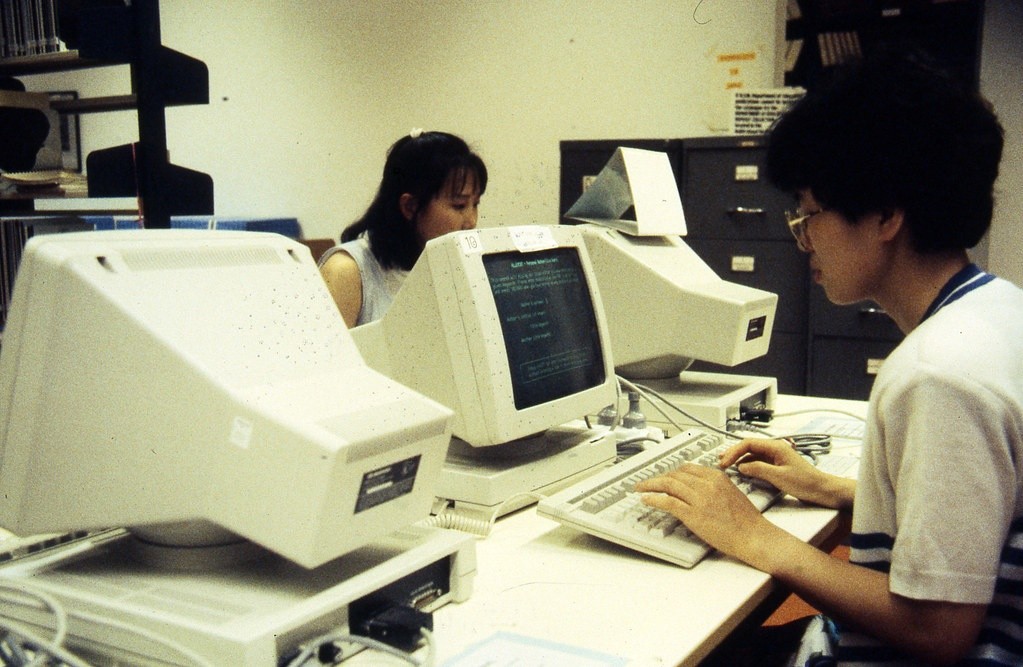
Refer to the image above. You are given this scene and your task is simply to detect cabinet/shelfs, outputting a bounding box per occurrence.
[556,137,908,404]
[0,1,217,233]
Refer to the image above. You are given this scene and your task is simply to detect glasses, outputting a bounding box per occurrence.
[785,206,829,252]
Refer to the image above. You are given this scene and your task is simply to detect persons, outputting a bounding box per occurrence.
[632,45,1022,666]
[315,128,489,331]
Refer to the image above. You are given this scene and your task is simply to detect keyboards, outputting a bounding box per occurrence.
[535,428,819,567]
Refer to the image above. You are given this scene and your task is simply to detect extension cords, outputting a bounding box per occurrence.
[562,420,663,453]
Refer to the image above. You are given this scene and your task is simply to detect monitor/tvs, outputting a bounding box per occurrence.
[3,228,451,562]
[347,225,618,443]
[569,223,777,380]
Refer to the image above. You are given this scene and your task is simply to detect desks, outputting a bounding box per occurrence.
[322,390,870,667]
